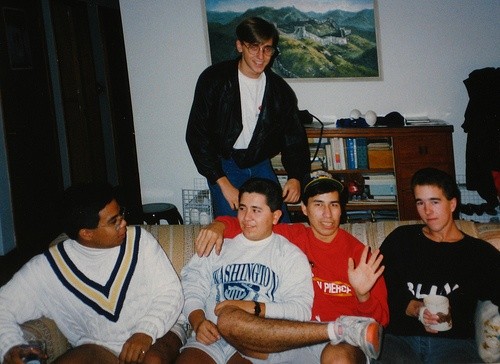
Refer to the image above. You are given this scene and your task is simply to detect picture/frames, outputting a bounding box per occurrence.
[201,0,382,81]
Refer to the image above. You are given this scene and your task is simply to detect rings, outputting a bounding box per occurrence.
[142,351,145,353]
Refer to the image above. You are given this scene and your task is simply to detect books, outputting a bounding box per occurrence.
[270,137,369,171]
[406,116,429,123]
[362,172,397,200]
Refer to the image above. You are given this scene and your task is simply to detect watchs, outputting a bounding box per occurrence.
[253,301,261,316]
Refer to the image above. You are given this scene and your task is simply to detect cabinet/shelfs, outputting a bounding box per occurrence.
[270,119,458,224]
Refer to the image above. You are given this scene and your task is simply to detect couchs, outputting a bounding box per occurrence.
[21,220,500,363]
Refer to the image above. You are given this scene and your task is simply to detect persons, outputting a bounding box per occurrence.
[0,180,184,364]
[186,17,311,224]
[371,166,500,364]
[195,176,391,364]
[180,178,314,364]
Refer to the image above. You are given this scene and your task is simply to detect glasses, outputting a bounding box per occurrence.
[243,42,275,57]
[86,209,127,232]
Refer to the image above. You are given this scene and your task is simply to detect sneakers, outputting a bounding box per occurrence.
[330,315,383,360]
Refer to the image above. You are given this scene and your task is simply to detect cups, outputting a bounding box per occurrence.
[424,295,452,331]
[19,340,47,364]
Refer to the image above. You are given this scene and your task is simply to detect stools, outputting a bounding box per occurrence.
[133,203,184,225]
